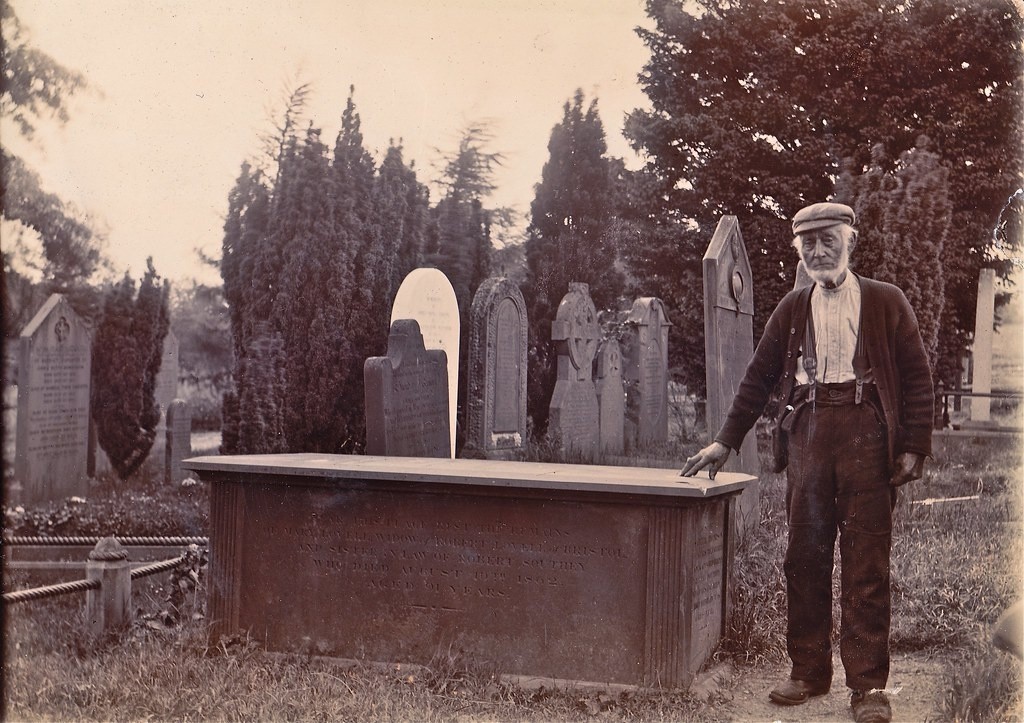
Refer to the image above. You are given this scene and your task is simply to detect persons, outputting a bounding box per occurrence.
[679,201,936,723]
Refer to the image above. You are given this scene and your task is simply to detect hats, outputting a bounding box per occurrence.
[792,202,855,236]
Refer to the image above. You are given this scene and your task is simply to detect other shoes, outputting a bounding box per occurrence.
[847,689,892,723]
[769,678,829,705]
